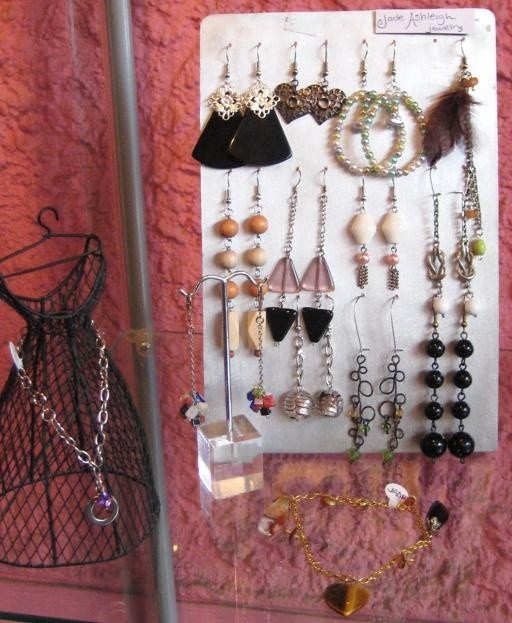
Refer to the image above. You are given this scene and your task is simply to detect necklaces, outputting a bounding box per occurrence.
[9,318,119,525]
[290,490,441,617]
